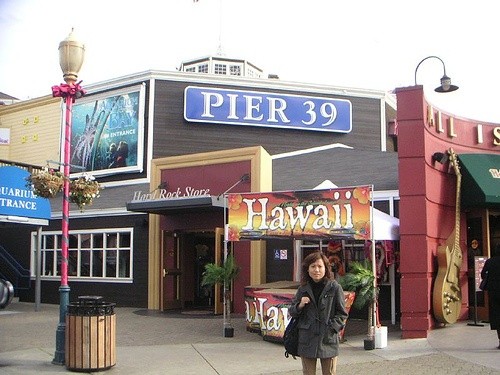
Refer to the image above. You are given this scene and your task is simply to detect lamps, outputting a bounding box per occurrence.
[414,55,459,93]
[432,151,449,164]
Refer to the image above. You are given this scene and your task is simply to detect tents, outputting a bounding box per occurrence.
[312,179,400,325]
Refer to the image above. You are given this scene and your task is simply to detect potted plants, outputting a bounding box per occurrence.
[199,253,242,338]
[338,257,380,350]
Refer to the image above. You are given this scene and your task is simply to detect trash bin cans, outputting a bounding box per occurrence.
[65,296,116,373]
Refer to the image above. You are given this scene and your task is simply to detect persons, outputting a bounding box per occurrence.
[288,250,348,375]
[468,239,484,306]
[479,242,500,350]
[107,141,128,168]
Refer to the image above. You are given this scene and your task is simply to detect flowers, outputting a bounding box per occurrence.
[25,168,101,210]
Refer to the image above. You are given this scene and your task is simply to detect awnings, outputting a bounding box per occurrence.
[456,154,500,203]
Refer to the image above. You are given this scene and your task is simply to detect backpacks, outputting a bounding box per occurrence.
[283,288,307,357]
